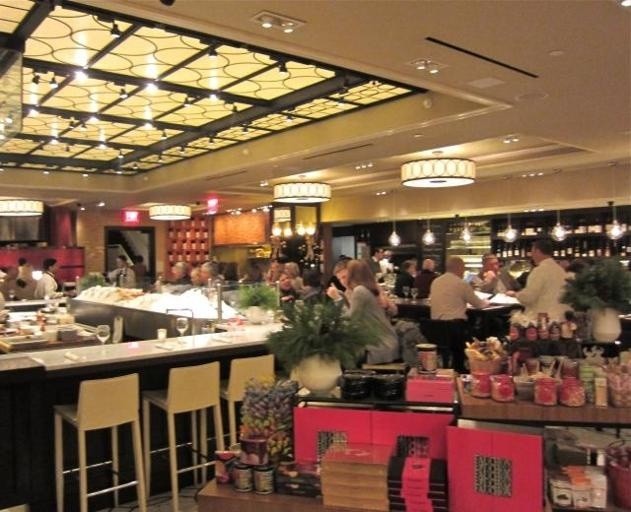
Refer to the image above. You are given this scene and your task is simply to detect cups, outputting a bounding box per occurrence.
[157,328,167,344]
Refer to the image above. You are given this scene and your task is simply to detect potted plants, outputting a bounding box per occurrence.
[229,282,279,324]
[266,287,393,395]
[558,256,631,345]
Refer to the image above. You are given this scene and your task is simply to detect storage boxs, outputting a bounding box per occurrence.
[405,375,457,404]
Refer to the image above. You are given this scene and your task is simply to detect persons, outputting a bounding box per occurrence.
[427,257,492,375]
[104,255,147,283]
[329,258,399,323]
[503,239,569,325]
[326,258,401,375]
[469,252,536,293]
[163,260,239,289]
[0,256,75,309]
[237,258,322,305]
[558,258,588,275]
[378,257,441,299]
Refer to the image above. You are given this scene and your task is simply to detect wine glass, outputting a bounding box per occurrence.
[175,318,189,343]
[227,315,241,337]
[410,288,419,304]
[95,324,111,354]
[261,309,287,332]
[402,286,410,301]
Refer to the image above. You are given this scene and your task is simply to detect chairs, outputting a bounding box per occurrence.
[201,352,280,487]
[51,371,142,512]
[140,360,224,510]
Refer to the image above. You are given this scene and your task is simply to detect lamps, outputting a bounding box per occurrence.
[400,157,477,189]
[460,218,474,244]
[605,162,626,241]
[405,57,450,75]
[273,182,332,206]
[421,192,436,247]
[502,176,518,243]
[0,199,46,217]
[388,188,403,247]
[149,205,192,222]
[550,166,568,242]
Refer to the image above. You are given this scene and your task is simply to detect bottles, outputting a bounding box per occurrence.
[495,219,631,235]
[471,355,610,408]
[448,222,491,232]
[494,239,631,258]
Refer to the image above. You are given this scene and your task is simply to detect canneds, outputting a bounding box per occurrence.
[233,462,253,493]
[253,464,276,495]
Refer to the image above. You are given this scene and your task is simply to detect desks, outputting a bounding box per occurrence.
[457,376,631,429]
[1,297,69,313]
[196,476,383,511]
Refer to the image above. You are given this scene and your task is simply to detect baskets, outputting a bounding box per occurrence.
[468,352,507,375]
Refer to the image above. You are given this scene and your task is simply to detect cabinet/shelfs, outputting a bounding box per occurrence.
[492,205,630,268]
